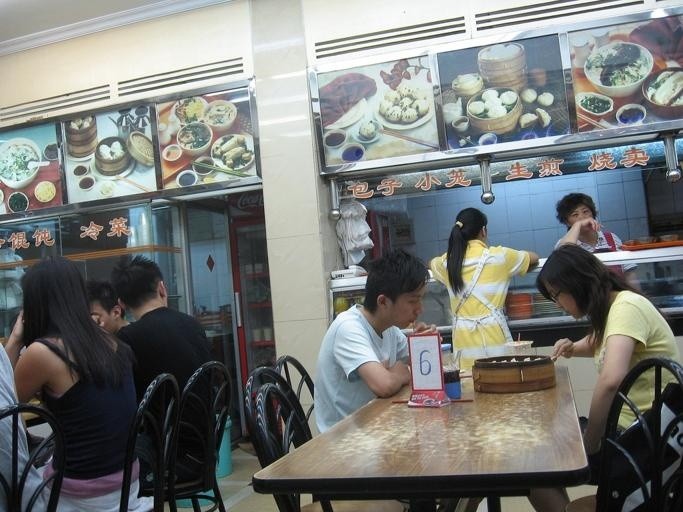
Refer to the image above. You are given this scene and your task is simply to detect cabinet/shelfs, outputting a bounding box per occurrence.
[338,243,681,349]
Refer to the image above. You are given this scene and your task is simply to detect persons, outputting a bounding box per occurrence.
[527,243,682,512]
[114,254,209,484]
[83,280,131,336]
[553,192,642,342]
[427,207,539,372]
[4,259,142,511]
[0,342,43,512]
[312,248,443,433]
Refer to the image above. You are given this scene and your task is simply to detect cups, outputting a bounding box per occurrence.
[573,36,588,64]
[503,340,532,356]
[591,27,609,48]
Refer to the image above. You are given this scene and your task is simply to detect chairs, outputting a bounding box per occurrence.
[569,353,682,512]
[267,349,322,443]
[252,377,406,512]
[106,370,181,512]
[240,354,333,512]
[128,348,234,512]
[0,399,65,511]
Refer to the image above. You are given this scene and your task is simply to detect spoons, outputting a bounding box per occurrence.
[24,159,51,170]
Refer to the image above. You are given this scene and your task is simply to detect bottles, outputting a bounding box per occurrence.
[168,115,179,136]
[158,122,171,147]
[438,344,461,398]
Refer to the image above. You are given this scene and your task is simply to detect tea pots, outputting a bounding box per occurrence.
[107,107,133,141]
[442,96,461,126]
[128,106,151,140]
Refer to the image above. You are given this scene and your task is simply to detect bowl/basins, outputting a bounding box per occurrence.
[613,103,645,124]
[621,232,678,244]
[201,101,236,129]
[452,114,469,131]
[0,137,41,189]
[176,122,212,153]
[477,131,496,146]
[582,41,653,96]
[521,133,535,140]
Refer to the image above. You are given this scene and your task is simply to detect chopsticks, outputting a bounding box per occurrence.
[114,173,152,193]
[381,130,440,150]
[188,160,250,177]
[458,135,474,146]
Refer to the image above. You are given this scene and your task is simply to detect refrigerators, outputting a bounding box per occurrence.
[226,191,392,439]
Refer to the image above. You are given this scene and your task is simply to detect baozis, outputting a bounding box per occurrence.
[451,43,555,128]
[70,115,93,130]
[98,141,125,161]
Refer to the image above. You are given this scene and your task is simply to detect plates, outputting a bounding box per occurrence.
[91,155,136,181]
[70,163,89,177]
[192,156,214,175]
[374,101,433,131]
[351,119,381,144]
[33,180,56,203]
[175,169,197,187]
[169,95,207,123]
[6,191,30,214]
[65,152,95,162]
[209,133,253,171]
[322,97,367,130]
[161,144,181,161]
[76,176,96,191]
[42,142,59,162]
[336,142,366,162]
[323,127,348,149]
[575,91,613,116]
